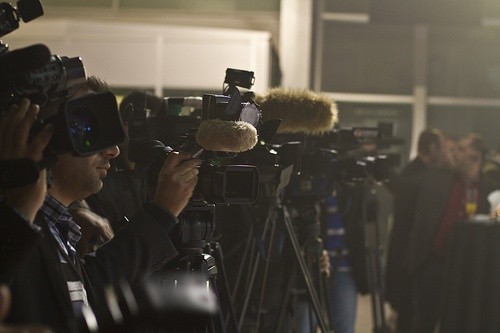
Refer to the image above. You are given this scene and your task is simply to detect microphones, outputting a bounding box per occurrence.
[254,87,339,133]
[187,118,259,154]
[1,43,51,85]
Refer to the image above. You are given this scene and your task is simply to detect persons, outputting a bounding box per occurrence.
[0,74,500,333]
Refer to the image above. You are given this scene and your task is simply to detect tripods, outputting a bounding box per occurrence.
[178,177,390,333]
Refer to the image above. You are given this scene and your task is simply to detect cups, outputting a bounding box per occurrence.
[465,189,477,217]
